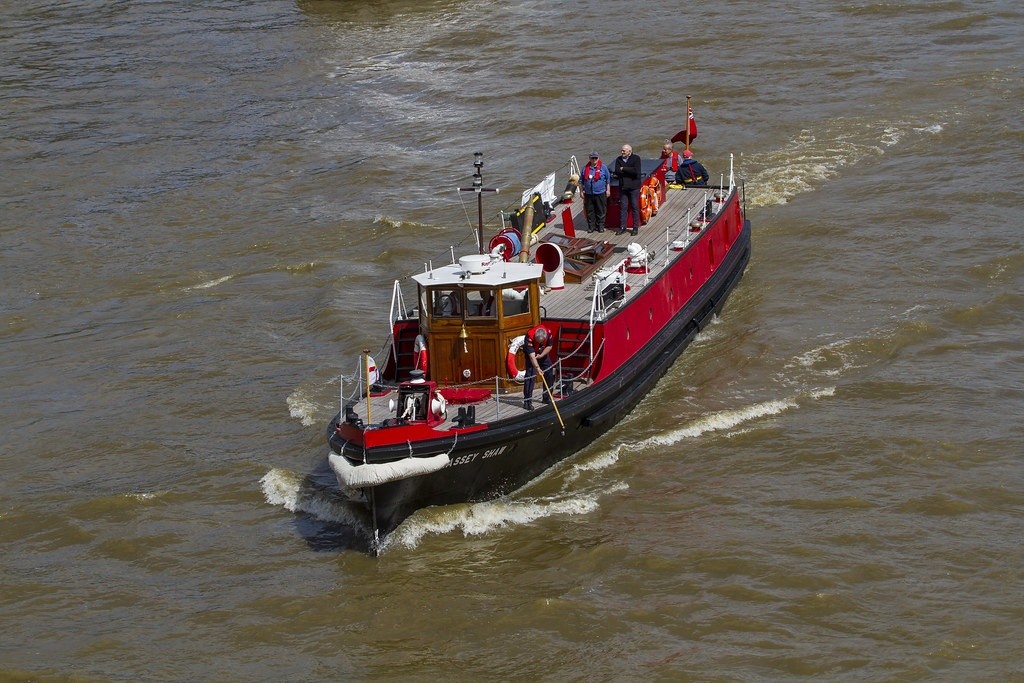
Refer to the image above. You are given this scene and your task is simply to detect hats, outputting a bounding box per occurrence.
[589,151,599,157]
[683,150,693,158]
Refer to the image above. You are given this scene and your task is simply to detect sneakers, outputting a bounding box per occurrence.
[542,398,552,404]
[524,403,534,410]
[595,225,604,233]
[588,226,596,233]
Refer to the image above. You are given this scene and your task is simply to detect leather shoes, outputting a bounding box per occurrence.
[631,228,638,236]
[615,226,626,235]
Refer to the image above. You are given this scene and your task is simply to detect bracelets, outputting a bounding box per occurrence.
[535,365,539,369]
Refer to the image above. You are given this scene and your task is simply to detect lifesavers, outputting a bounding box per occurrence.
[413,334,428,379]
[506,335,527,381]
[637,176,663,225]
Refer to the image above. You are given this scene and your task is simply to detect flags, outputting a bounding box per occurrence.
[672,107,697,145]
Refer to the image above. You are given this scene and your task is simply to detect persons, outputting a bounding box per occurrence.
[476,291,493,316]
[444,291,475,315]
[615,145,642,235]
[580,152,610,231]
[676,152,708,185]
[523,325,553,411]
[660,142,681,177]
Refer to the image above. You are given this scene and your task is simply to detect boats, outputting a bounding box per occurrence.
[326,93,752,556]
